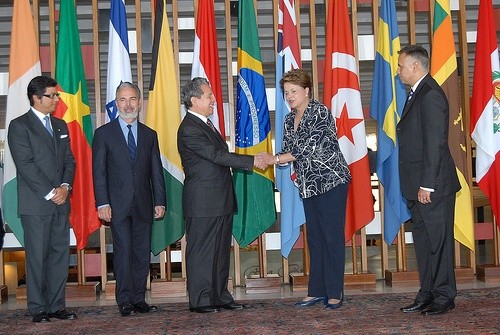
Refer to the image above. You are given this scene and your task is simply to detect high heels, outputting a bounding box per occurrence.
[324,297,343,310]
[294,296,327,308]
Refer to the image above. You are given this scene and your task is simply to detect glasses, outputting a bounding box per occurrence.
[42,92,59,99]
[116,97,138,103]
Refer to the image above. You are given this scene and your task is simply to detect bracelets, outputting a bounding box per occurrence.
[277,154,280,165]
[63,184,72,190]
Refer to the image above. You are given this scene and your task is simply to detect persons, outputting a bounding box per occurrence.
[176,77,269,313]
[256,67,353,310]
[8,76,78,322]
[90,80,168,319]
[396,46,462,316]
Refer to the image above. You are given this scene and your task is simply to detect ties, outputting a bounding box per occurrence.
[127,125,136,166]
[44,116,53,138]
[207,119,218,136]
[405,89,414,107]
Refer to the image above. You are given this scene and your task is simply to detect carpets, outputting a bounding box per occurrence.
[0,285,500,335]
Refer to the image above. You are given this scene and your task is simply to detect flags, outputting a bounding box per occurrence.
[372,0,411,245]
[0,0,78,248]
[104,0,133,125]
[143,0,187,256]
[324,0,374,243]
[470,0,500,229]
[191,0,226,140]
[52,0,102,250]
[429,0,475,253]
[271,0,306,260]
[233,0,278,249]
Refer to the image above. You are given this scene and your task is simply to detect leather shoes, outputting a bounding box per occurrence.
[32,312,50,323]
[48,309,76,320]
[400,299,429,313]
[421,302,456,316]
[213,301,246,311]
[189,305,223,313]
[135,301,157,314]
[120,305,138,317]
